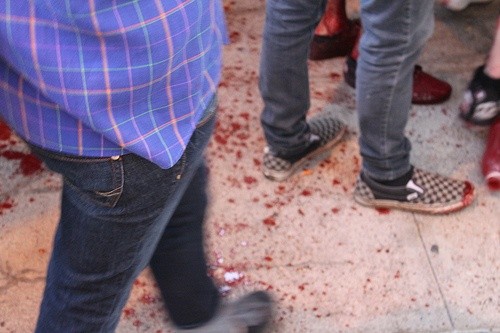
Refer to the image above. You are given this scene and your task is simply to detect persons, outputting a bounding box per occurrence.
[0,0,275,333]
[259,0,500,213]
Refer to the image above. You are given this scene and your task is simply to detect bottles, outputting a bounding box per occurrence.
[481,117,500,191]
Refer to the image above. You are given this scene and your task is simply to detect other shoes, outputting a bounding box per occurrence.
[457,64,500,126]
[170,289,274,333]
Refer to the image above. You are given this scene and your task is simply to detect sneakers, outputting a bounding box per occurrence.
[261,116,346,183]
[342,54,452,105]
[308,17,361,61]
[353,165,476,215]
